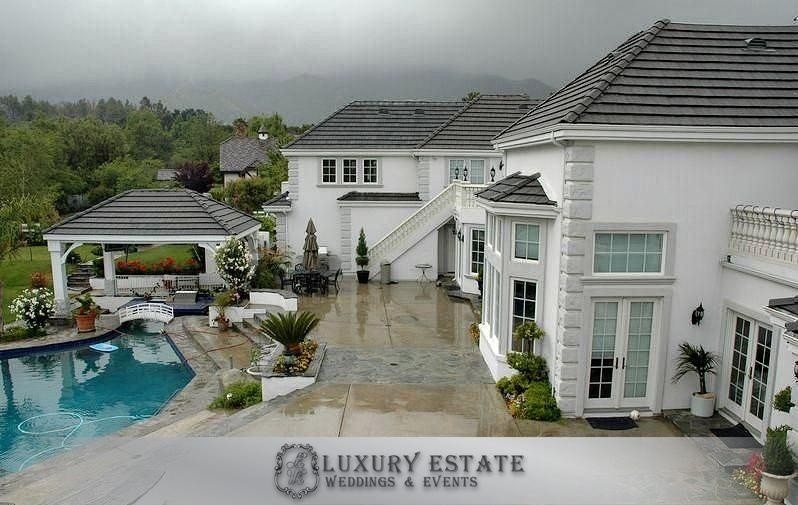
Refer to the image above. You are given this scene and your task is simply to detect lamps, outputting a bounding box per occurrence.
[691,302,704,326]
[793,360,798,384]
[463,166,468,180]
[454,166,459,180]
[490,165,495,182]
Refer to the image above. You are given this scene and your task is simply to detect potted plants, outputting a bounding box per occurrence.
[257,310,320,356]
[355,225,369,284]
[71,293,101,333]
[669,341,721,418]
[209,289,232,332]
[754,425,797,505]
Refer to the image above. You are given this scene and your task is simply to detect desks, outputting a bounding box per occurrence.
[415,265,432,285]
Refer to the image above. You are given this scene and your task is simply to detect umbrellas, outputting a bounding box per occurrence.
[303,218,319,270]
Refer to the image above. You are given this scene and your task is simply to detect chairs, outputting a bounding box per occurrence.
[291,262,341,296]
